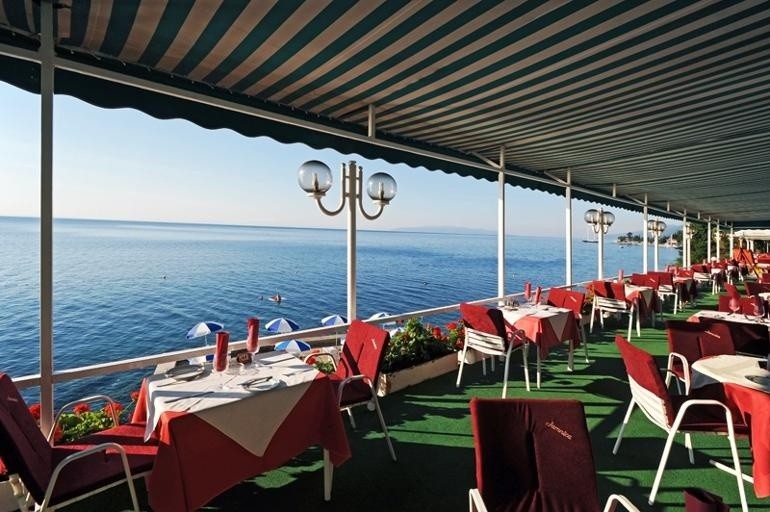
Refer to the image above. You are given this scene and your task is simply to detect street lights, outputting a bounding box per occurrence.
[686,221,698,268]
[583,207,617,280]
[647,218,667,275]
[297,158,399,326]
[712,229,726,263]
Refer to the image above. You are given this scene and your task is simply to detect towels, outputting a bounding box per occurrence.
[216,316,260,371]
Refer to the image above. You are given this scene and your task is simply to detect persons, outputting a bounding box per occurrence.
[275,293,281,302]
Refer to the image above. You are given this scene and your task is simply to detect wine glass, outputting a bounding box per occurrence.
[212,352,229,380]
[525,294,532,307]
[753,304,766,324]
[245,335,260,369]
[729,299,741,319]
[674,271,680,278]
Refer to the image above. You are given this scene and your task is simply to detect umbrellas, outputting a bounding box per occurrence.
[274,339,311,352]
[369,311,396,330]
[265,317,299,333]
[321,314,348,346]
[189,354,214,365]
[186,320,224,346]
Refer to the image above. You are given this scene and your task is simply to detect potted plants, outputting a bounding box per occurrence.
[373,313,461,398]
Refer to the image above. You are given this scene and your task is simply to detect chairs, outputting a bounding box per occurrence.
[304,317,400,464]
[0,371,159,512]
[455,247,769,512]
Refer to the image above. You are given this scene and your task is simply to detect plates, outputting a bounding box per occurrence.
[743,373,770,390]
[242,375,280,392]
[164,364,205,382]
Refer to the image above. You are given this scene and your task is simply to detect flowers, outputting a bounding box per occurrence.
[432,316,470,351]
[28,388,142,445]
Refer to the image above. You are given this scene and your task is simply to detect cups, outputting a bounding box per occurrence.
[534,298,541,308]
